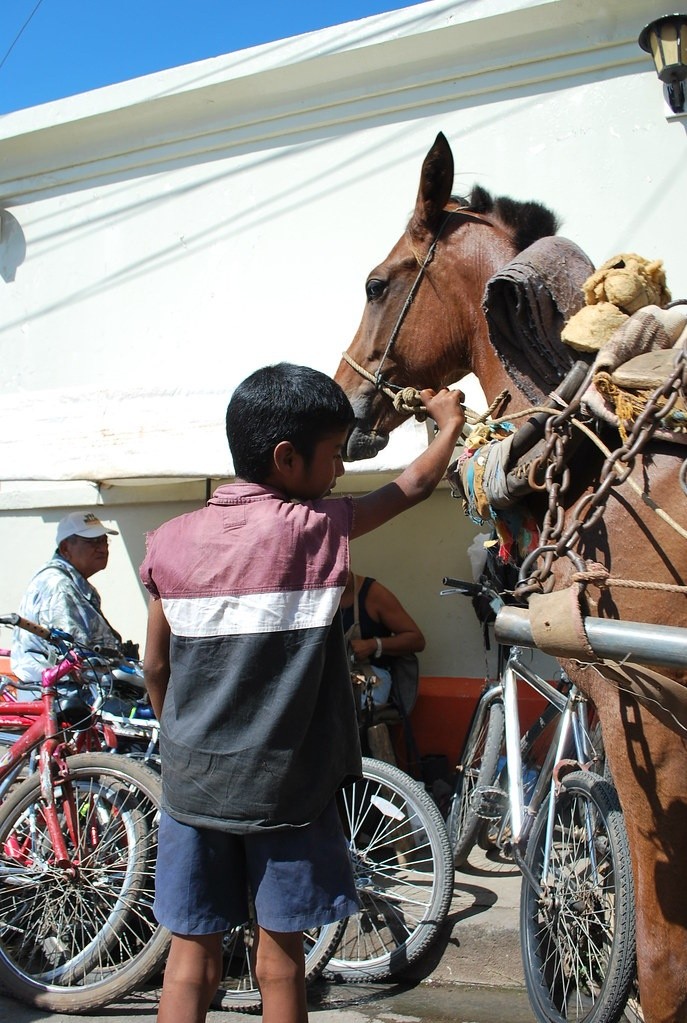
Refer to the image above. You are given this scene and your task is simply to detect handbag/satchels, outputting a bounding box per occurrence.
[341,622,381,685]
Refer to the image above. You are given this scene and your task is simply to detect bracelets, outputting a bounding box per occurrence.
[373,637,382,658]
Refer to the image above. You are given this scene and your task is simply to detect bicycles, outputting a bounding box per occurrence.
[431,543,648,1023]
[0,613,452,1015]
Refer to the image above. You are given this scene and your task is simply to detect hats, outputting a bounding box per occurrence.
[57,512,119,547]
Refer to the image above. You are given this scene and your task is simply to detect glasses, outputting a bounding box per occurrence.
[71,536,109,545]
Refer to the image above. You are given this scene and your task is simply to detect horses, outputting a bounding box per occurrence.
[330,131,687,1023]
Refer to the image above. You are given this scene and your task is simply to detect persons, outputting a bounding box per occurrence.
[140,363,465,1023]
[338,567,426,866]
[10,511,161,829]
